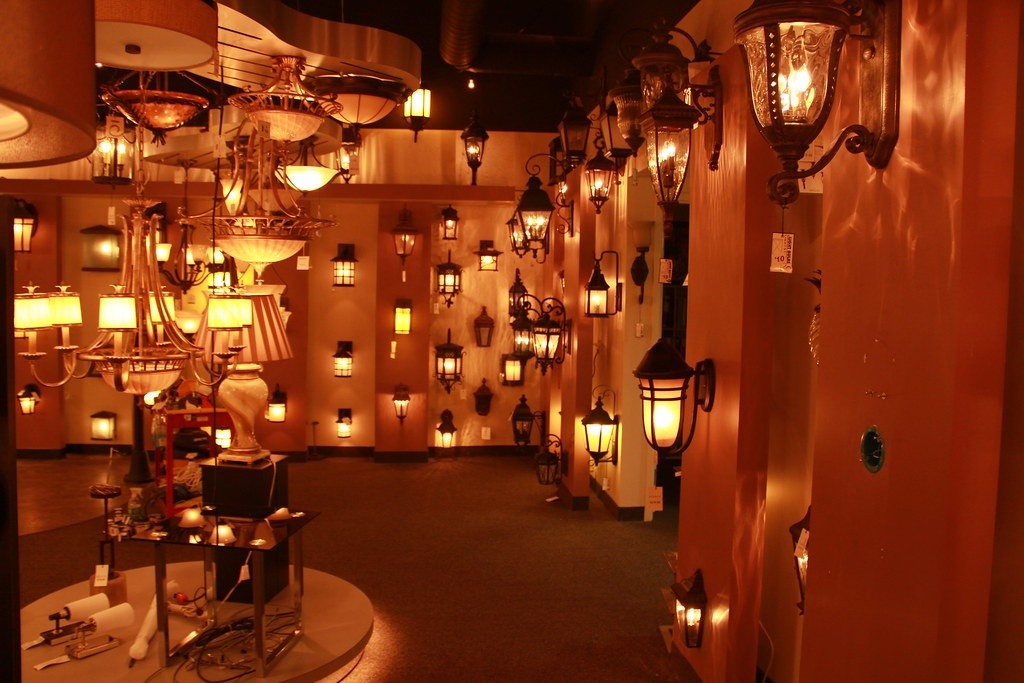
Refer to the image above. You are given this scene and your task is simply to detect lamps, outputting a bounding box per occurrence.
[0,0,899,683]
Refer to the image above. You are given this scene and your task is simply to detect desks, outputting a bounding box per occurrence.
[100,492,319,679]
[20,562,375,683]
